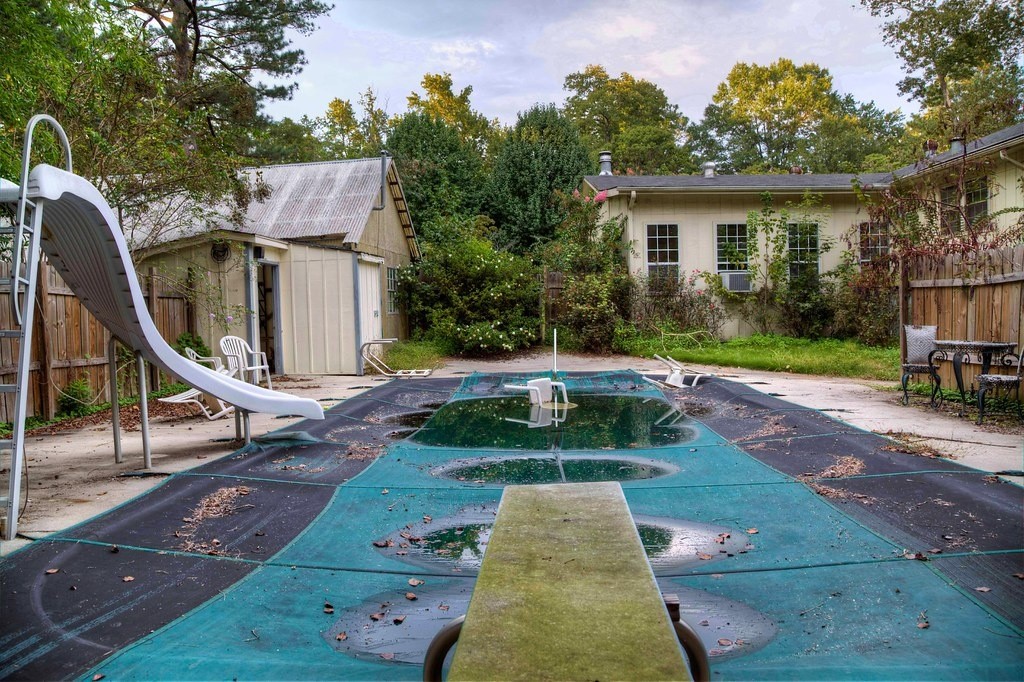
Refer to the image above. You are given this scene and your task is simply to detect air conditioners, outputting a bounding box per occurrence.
[718,272,752,293]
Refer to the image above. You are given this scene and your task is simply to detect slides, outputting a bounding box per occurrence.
[0,164,326,421]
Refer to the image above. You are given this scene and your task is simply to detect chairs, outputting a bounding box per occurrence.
[505,405,568,428]
[642,353,712,388]
[185,346,224,402]
[900,324,941,407]
[503,376,578,410]
[220,336,273,390]
[642,399,684,426]
[973,344,1024,426]
[158,364,239,421]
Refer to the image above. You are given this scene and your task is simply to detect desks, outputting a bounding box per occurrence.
[926,339,1018,416]
